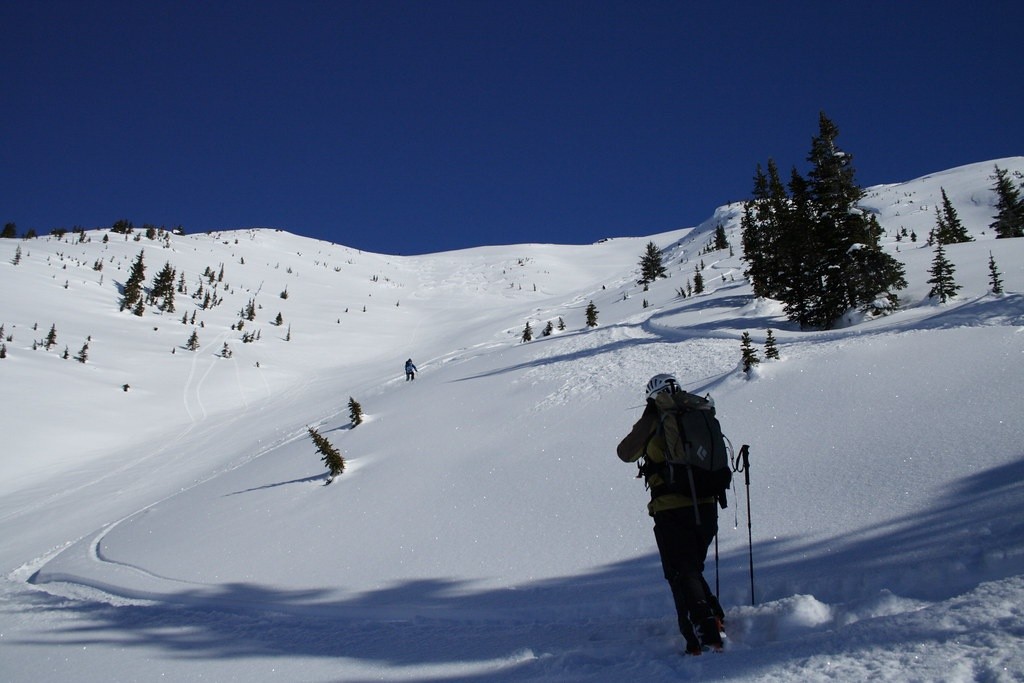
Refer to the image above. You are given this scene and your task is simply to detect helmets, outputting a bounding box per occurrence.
[645,373,681,403]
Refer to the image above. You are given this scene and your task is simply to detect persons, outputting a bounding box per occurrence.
[617,373,732,658]
[405,359,417,381]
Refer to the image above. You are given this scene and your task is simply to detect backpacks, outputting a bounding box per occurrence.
[645,391,733,509]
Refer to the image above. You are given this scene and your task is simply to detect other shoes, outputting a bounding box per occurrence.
[715,615,726,638]
[682,642,724,657]
